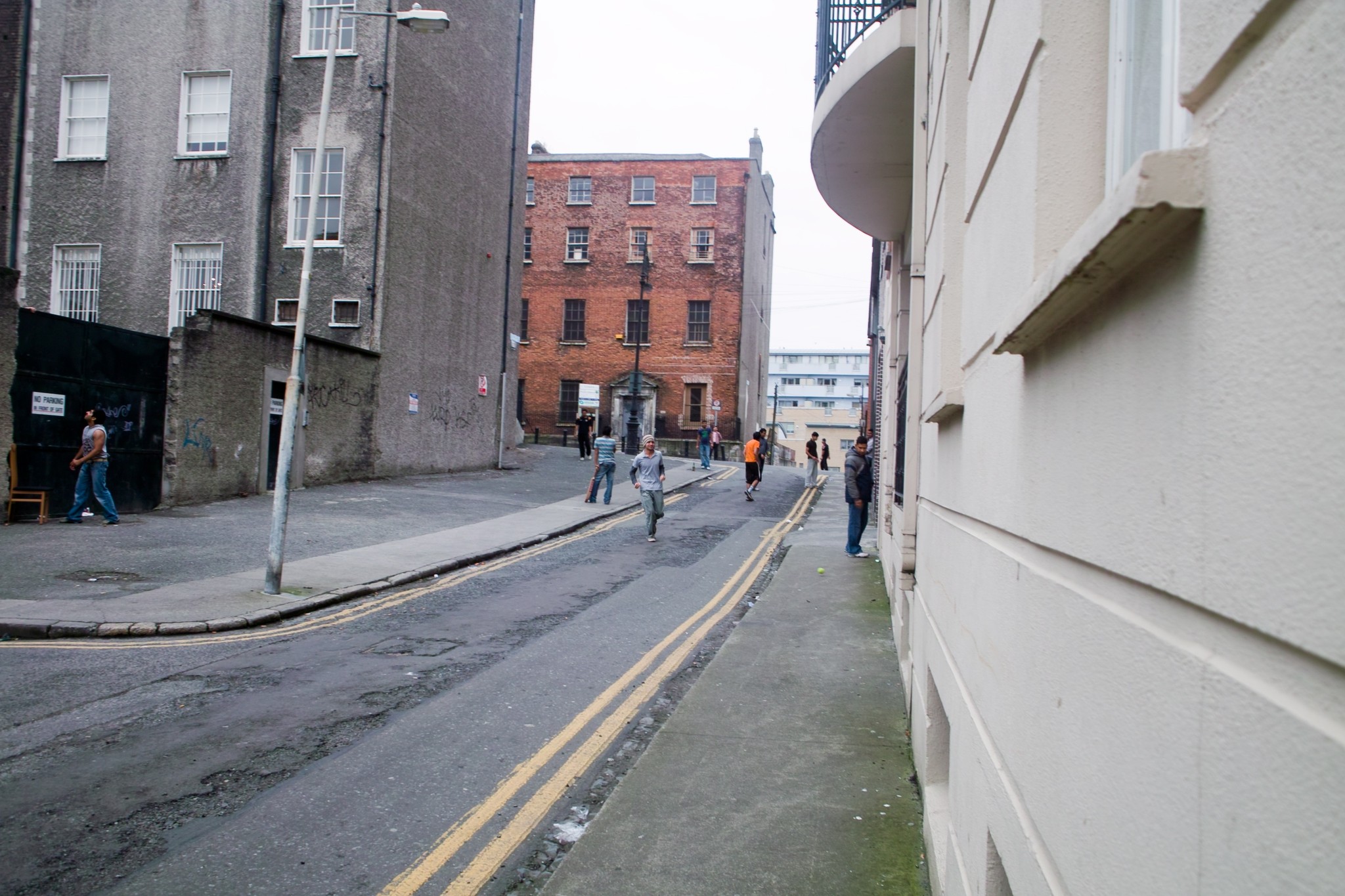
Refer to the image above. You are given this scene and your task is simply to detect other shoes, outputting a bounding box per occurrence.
[707,466,710,470]
[101,519,120,525]
[648,537,656,542]
[588,455,592,460]
[581,457,584,460]
[804,485,813,488]
[60,518,83,524]
[754,488,760,491]
[744,491,754,501]
[812,485,819,488]
[701,466,705,469]
[844,548,870,557]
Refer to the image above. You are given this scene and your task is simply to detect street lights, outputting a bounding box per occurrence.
[263,5,456,596]
[624,232,655,456]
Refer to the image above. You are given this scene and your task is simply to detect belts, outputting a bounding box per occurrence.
[88,458,108,463]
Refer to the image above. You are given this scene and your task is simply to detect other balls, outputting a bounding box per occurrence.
[818,568,825,574]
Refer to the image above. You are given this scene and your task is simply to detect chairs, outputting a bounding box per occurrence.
[3,443,52,526]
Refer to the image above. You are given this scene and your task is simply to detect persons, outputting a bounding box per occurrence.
[59,408,121,525]
[574,409,593,460]
[806,432,819,488]
[820,439,831,471]
[845,435,873,557]
[865,429,874,469]
[750,428,770,491]
[743,431,761,502]
[696,420,713,470]
[584,426,617,505]
[710,426,723,461]
[630,434,666,542]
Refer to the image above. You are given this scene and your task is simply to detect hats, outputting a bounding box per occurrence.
[643,434,656,448]
